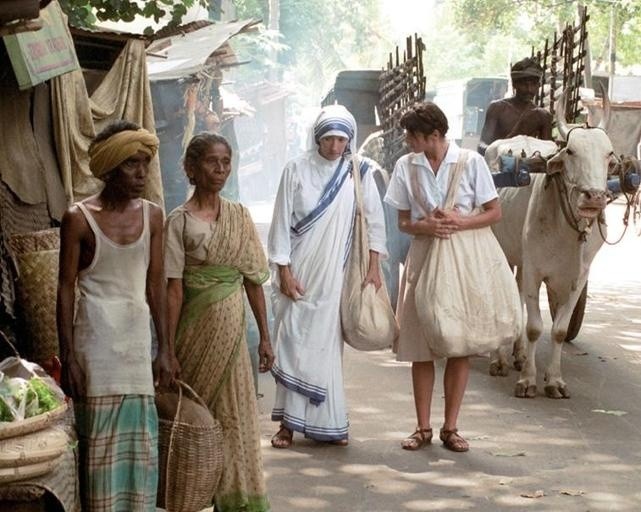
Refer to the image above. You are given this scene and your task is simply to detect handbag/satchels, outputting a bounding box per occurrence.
[415,203,525,358]
[334,214,403,356]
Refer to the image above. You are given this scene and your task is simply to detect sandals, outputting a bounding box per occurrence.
[439,427,470,452]
[401,426,433,450]
[271,424,293,448]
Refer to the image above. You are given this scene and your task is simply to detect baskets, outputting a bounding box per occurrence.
[152,377,224,512]
[8,228,75,360]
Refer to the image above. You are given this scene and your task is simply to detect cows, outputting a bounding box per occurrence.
[476,80,613,399]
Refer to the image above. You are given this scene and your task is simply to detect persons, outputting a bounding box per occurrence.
[55,119,176,512]
[476,55,554,159]
[161,132,277,512]
[381,100,504,453]
[266,104,391,448]
